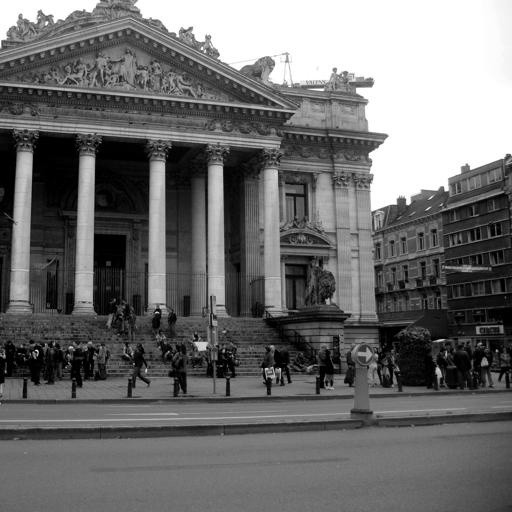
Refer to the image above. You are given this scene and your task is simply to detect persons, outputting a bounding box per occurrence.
[260,346,314,385]
[342,70,353,92]
[318,346,356,389]
[105,299,178,339]
[304,257,318,305]
[123,343,152,387]
[366,347,402,392]
[0,339,110,387]
[329,67,340,92]
[15,8,223,99]
[424,341,511,390]
[157,330,238,395]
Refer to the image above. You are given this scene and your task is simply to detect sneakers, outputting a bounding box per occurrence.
[325,385,335,390]
